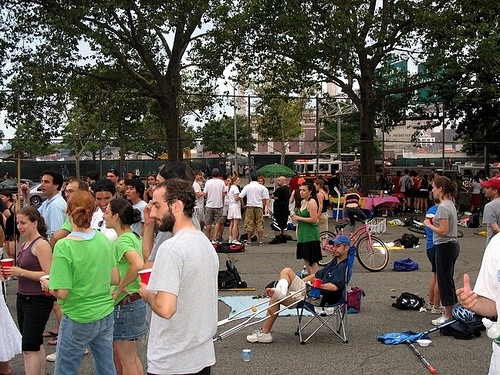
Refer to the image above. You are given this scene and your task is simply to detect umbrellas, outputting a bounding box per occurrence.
[257,163,296,192]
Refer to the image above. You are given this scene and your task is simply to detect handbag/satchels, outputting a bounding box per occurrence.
[218,259,247,289]
[392,291,425,310]
[346,286,366,314]
[213,243,246,252]
[439,317,481,339]
[397,233,419,248]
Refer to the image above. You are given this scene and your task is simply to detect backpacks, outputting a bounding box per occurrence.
[393,258,418,271]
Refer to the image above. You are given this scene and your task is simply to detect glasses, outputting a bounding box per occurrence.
[96,219,103,231]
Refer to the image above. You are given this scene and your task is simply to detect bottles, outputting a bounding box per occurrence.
[391,289,396,298]
[481,317,500,347]
[300,267,308,278]
[289,207,299,226]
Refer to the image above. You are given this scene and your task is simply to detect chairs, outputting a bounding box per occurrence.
[295,246,358,344]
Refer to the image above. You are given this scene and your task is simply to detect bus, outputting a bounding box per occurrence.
[291,159,343,181]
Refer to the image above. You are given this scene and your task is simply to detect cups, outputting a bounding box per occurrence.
[426,214,435,225]
[243,349,251,361]
[313,279,323,288]
[41,274,53,296]
[0,258,14,277]
[138,269,152,285]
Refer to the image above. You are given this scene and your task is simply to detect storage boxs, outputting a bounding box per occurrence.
[333,208,347,218]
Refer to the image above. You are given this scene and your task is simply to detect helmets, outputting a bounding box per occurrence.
[452,304,476,320]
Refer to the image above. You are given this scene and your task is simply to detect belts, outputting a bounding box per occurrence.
[117,293,143,305]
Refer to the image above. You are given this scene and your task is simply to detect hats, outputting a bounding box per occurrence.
[480,177,500,190]
[329,235,350,245]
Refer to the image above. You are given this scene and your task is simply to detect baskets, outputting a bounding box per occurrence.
[367,217,386,235]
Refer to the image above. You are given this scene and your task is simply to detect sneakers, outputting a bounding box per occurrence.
[246,329,273,342]
[211,231,288,246]
[426,302,435,309]
[431,315,450,326]
[431,304,446,314]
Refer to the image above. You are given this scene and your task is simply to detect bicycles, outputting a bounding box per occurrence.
[317,212,389,272]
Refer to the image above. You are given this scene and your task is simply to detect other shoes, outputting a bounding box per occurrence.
[399,208,423,213]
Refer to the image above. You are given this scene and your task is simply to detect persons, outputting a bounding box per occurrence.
[0,158,500,375]
[246,234,351,344]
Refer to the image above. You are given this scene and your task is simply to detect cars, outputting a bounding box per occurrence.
[0,178,34,193]
[29,182,48,206]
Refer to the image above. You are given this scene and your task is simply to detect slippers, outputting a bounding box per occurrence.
[43,329,89,361]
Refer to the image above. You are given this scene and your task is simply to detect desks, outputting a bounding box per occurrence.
[330,196,399,220]
